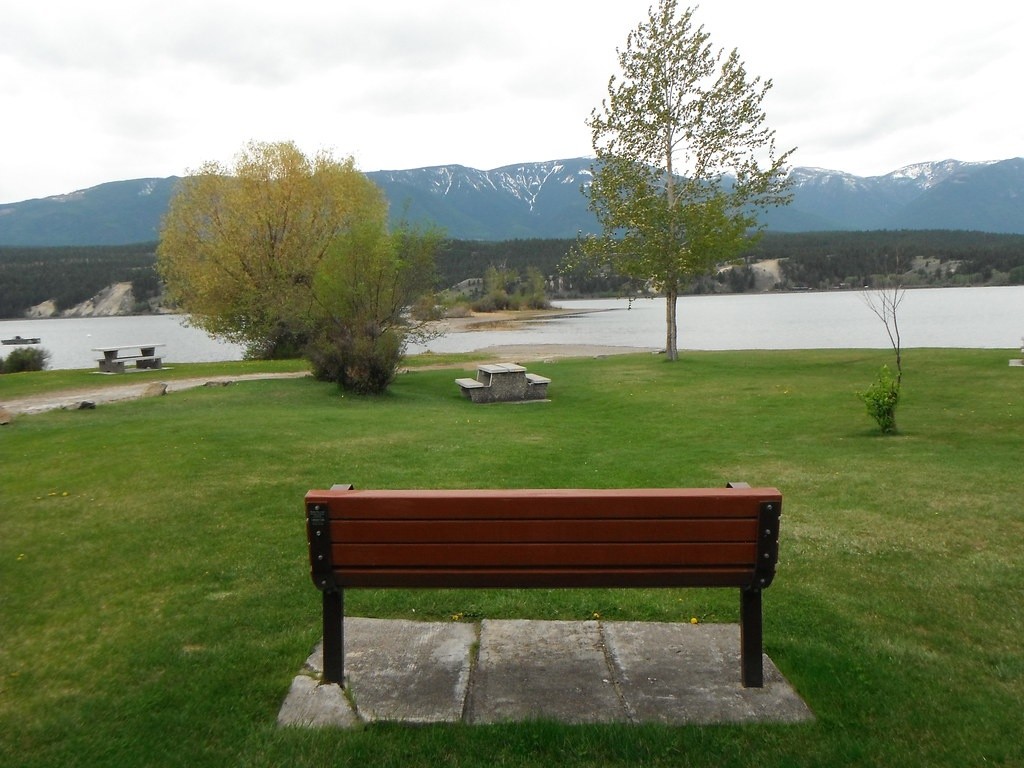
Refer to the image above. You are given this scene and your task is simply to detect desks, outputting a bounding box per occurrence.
[91,344,167,373]
[476,363,527,403]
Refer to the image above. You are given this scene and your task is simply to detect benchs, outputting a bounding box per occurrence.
[96,356,167,373]
[455,378,485,403]
[305,481,783,690]
[525,374,552,401]
